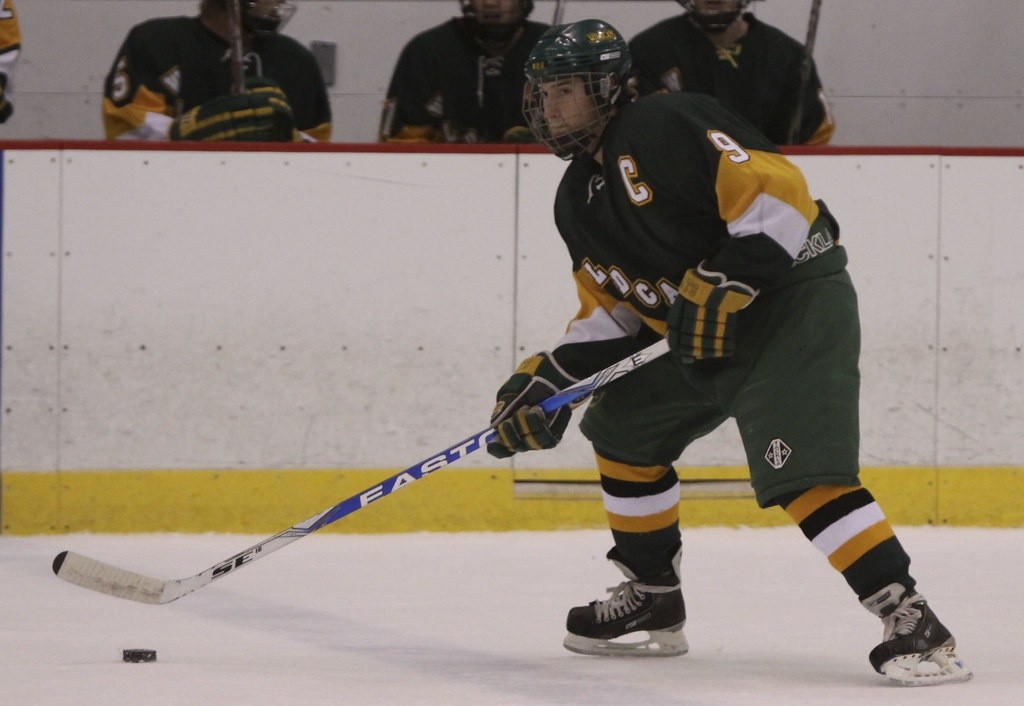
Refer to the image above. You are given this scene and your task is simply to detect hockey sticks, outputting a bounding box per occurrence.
[787,0,823,146]
[226,0,246,96]
[49,309,759,607]
[551,0,565,28]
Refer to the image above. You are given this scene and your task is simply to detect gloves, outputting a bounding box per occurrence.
[665,271,754,373]
[167,82,295,140]
[485,350,581,462]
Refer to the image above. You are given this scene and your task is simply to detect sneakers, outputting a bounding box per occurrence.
[856,579,972,683]
[563,548,689,656]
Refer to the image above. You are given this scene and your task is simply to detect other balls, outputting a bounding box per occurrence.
[121,648,157,664]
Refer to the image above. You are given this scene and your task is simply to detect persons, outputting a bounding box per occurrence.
[627,0,836,145]
[376,0,553,143]
[485,17,965,684]
[102,0,333,142]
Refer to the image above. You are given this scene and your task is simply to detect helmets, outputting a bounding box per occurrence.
[676,0,751,33]
[522,20,635,160]
[239,0,297,36]
[460,0,534,39]
